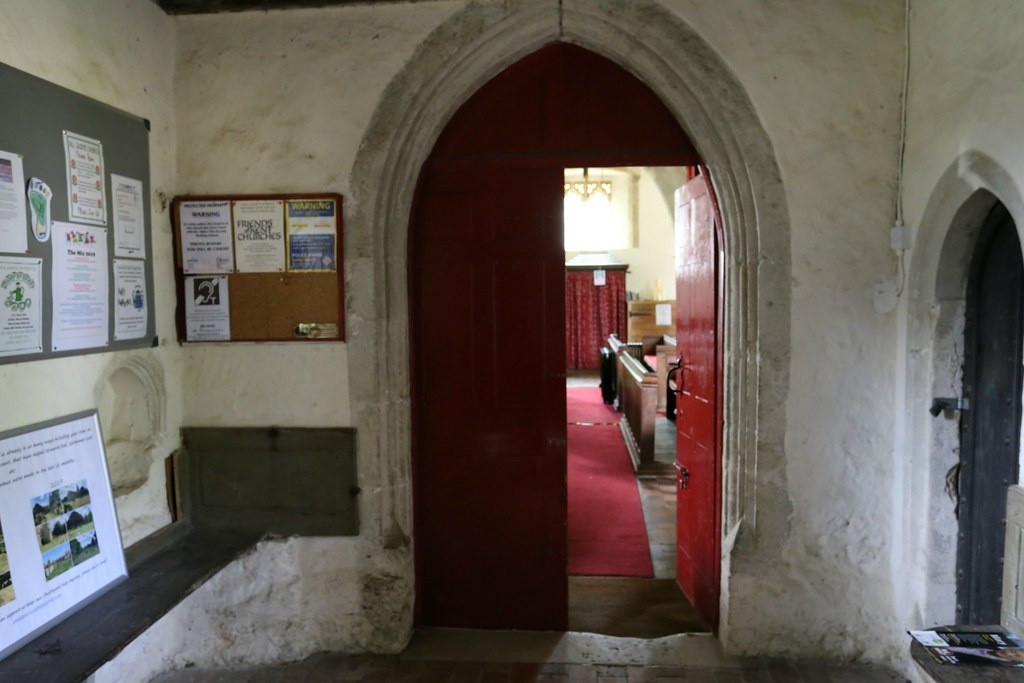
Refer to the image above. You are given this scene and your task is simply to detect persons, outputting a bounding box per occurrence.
[934,646,1024,667]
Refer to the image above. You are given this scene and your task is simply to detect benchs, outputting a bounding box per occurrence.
[643,334,679,411]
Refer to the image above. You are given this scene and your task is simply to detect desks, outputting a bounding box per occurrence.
[0,521,268,683]
